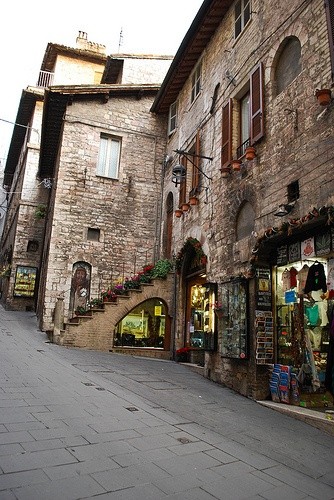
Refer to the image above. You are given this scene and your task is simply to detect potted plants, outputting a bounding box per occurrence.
[316,88,332,106]
[244,146,256,160]
[189,198,198,206]
[219,168,231,178]
[232,160,243,170]
[175,210,181,217]
[180,204,189,211]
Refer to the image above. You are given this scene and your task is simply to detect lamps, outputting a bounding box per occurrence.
[274,203,294,216]
[170,149,214,187]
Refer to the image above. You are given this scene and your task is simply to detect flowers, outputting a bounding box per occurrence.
[74,240,207,315]
